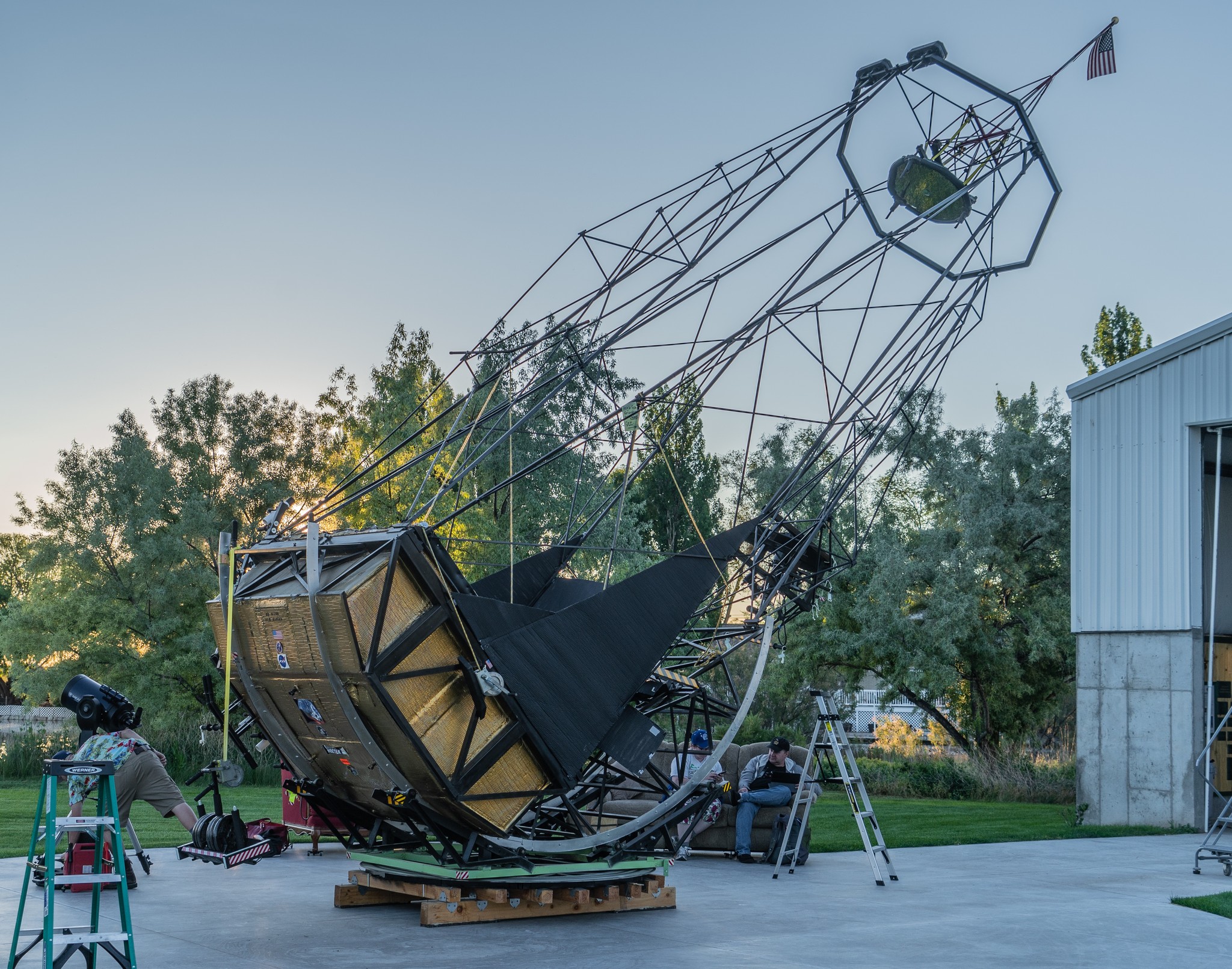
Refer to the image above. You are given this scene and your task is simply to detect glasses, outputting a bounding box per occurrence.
[696,745,709,751]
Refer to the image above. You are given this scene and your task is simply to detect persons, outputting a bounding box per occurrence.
[670,729,724,861]
[729,736,823,864]
[52,729,199,889]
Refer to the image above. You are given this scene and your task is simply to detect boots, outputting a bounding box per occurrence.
[103,857,138,890]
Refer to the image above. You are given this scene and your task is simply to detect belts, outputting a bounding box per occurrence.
[134,745,151,755]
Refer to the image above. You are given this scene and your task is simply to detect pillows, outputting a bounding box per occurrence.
[598,762,644,800]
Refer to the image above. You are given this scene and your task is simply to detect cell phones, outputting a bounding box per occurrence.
[713,771,727,777]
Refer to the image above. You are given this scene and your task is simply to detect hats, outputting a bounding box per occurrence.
[765,736,790,752]
[51,750,72,784]
[692,729,716,748]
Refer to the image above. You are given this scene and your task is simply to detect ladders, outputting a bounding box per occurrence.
[773,690,900,887]
[8,759,140,968]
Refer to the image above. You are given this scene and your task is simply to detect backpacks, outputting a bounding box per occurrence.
[764,814,811,866]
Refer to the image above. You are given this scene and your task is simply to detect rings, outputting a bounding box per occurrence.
[718,779,719,780]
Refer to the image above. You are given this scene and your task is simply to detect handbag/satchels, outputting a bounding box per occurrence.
[659,776,673,803]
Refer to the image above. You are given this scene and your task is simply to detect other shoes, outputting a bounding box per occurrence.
[728,789,741,807]
[739,853,755,864]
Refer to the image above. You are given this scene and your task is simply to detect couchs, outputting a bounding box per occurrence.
[588,740,818,859]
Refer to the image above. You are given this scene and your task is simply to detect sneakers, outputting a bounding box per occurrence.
[673,846,692,861]
[671,835,679,848]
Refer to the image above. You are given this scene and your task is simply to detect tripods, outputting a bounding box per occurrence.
[35,736,151,875]
